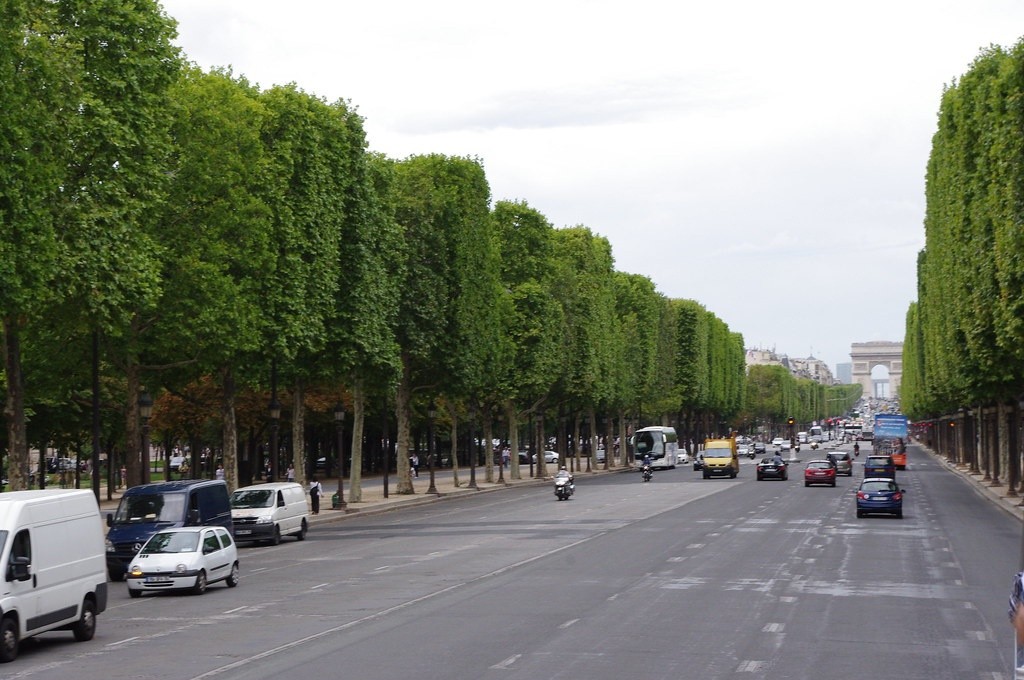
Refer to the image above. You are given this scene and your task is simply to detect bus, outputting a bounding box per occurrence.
[839,425,863,435]
[630,426,679,471]
[809,426,823,443]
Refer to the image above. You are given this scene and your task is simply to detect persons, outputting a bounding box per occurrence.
[771,451,783,464]
[178,462,189,479]
[554,465,574,494]
[854,442,859,452]
[796,440,800,451]
[409,453,419,478]
[502,446,511,468]
[216,462,224,480]
[309,476,325,515]
[915,434,920,442]
[120,465,126,487]
[746,443,757,457]
[264,456,294,482]
[641,455,653,477]
[891,438,905,455]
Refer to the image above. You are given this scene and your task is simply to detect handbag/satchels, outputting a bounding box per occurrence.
[310,486,319,496]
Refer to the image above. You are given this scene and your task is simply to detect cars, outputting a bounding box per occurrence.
[678,448,704,471]
[494,451,560,464]
[826,452,855,476]
[122,525,240,596]
[735,396,902,455]
[853,477,906,518]
[757,456,789,481]
[803,460,838,487]
[46,456,90,473]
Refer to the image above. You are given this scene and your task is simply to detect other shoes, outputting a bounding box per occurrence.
[312,512,314,514]
[649,474,653,476]
[315,512,318,514]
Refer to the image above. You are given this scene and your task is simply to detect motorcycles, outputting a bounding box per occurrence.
[794,445,800,452]
[749,451,755,459]
[642,464,653,482]
[811,444,818,450]
[854,447,859,456]
[550,476,575,501]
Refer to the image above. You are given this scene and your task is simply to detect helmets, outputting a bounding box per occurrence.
[560,466,566,470]
[644,455,649,459]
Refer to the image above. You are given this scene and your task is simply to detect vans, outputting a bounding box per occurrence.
[229,482,310,544]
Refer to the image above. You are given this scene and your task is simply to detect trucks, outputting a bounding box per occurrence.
[105,479,234,582]
[0,490,108,668]
[169,446,207,470]
[703,438,739,479]
[872,412,908,441]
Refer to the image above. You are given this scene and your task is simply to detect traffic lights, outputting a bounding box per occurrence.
[788,419,794,426]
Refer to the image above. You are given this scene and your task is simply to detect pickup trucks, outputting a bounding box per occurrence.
[873,439,906,470]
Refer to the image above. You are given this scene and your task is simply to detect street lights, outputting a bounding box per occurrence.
[332,401,348,510]
[135,386,154,484]
[269,403,281,483]
